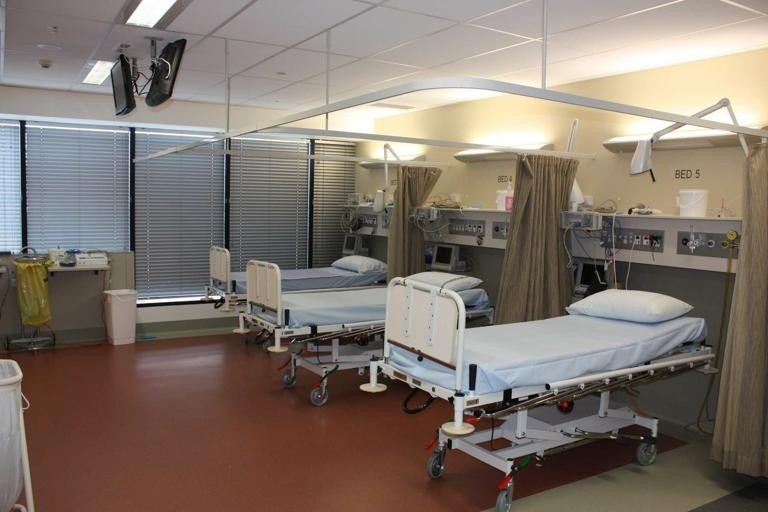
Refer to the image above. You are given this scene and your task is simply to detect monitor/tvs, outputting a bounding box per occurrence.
[145,39,188,106]
[110,55,137,116]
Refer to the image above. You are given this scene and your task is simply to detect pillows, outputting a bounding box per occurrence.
[407,270,485,292]
[331,254,389,276]
[564,288,696,324]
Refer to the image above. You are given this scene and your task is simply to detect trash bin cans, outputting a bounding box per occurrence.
[104,289,137,346]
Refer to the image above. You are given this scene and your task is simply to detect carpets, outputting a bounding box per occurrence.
[484,441,758,511]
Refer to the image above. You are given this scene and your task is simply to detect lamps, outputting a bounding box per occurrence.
[600,125,766,159]
[360,159,440,168]
[453,142,556,163]
[372,143,401,213]
[628,97,750,175]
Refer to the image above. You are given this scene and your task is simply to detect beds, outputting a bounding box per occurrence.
[383,277,719,511]
[247,258,496,407]
[207,244,389,342]
